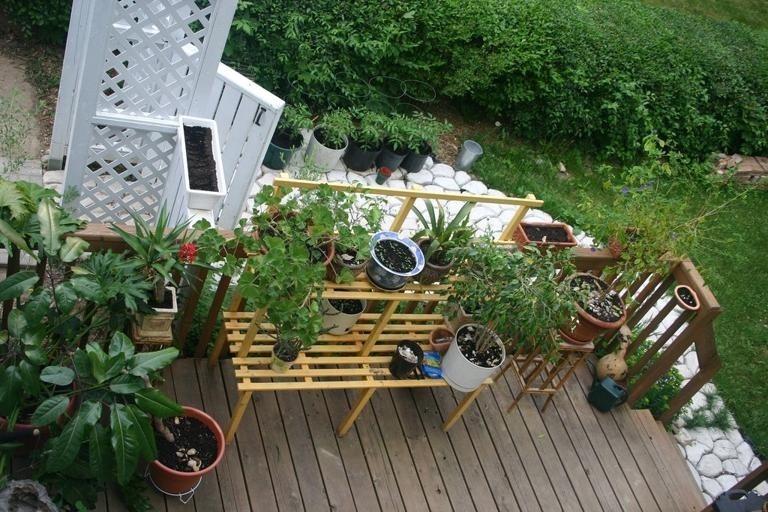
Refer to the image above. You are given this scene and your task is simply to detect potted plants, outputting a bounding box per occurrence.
[440,243,579,390]
[555,184,755,340]
[244,300,342,375]
[105,199,200,331]
[2,180,84,430]
[239,182,384,310]
[318,286,365,336]
[263,98,455,185]
[34,332,228,495]
[401,200,477,286]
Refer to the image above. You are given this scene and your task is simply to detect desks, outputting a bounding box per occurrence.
[496,324,595,414]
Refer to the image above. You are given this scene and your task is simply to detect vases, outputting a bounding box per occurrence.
[365,232,427,292]
[512,223,577,253]
[390,339,423,377]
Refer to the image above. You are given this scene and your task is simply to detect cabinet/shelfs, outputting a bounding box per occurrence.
[210,268,495,450]
